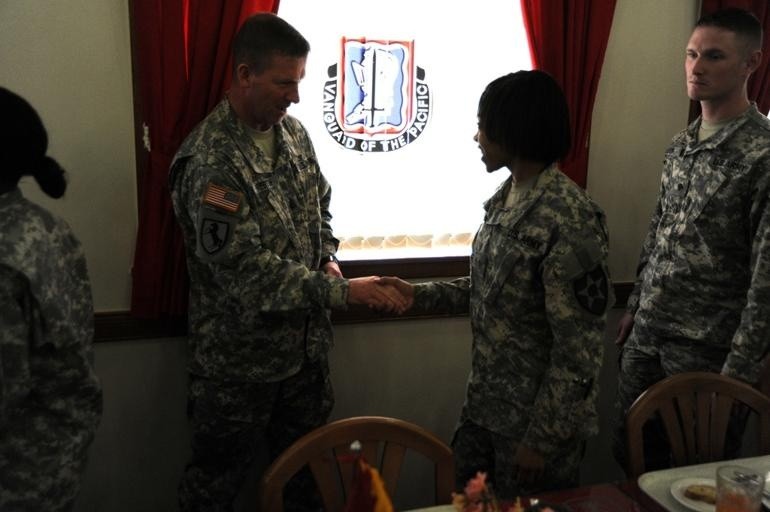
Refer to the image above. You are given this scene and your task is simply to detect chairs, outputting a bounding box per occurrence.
[627,370,770,478]
[258,416,457,511]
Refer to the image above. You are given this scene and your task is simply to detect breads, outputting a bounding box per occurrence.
[685,484,718,504]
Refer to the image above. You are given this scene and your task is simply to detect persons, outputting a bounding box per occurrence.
[0,88,102,511]
[363,70,619,500]
[167,11,410,512]
[609,8,770,483]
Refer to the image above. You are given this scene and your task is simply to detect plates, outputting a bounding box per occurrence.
[671,477,718,512]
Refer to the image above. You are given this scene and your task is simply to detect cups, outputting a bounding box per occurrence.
[715,464,765,512]
